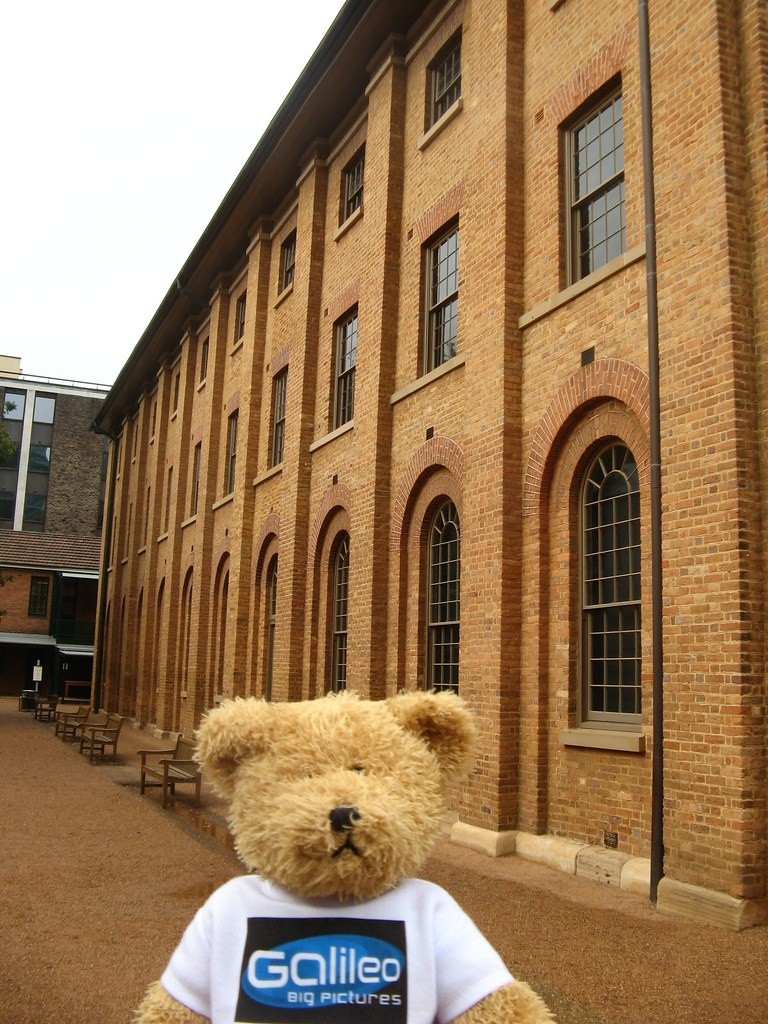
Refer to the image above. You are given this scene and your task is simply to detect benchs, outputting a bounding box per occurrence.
[34,695,58,722]
[137,733,202,808]
[54,703,91,741]
[78,713,124,761]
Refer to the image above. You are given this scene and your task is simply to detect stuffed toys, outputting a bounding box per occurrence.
[135,687,556,1024]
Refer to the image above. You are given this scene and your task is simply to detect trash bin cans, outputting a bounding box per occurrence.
[18,690,38,712]
